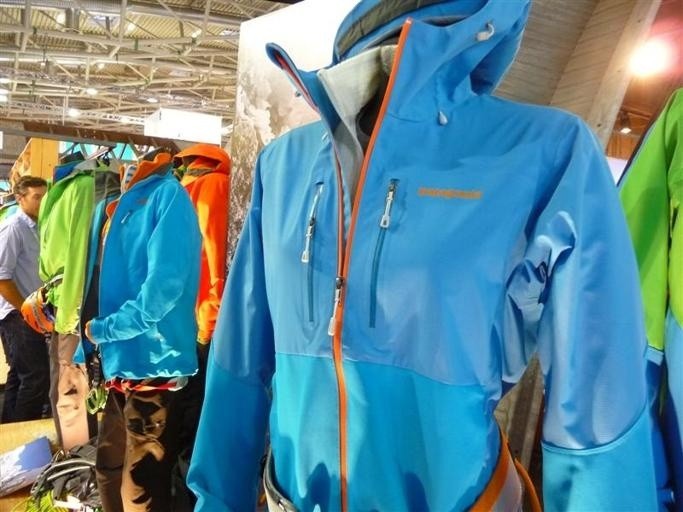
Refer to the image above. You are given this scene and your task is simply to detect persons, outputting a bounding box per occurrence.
[175,1,657,511]
[171,145,232,508]
[35,149,102,453]
[0,176,47,425]
[79,145,202,507]
[614,87,683,507]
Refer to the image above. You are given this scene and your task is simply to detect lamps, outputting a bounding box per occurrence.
[620,111,632,134]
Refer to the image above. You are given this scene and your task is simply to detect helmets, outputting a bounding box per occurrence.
[21,286,54,333]
